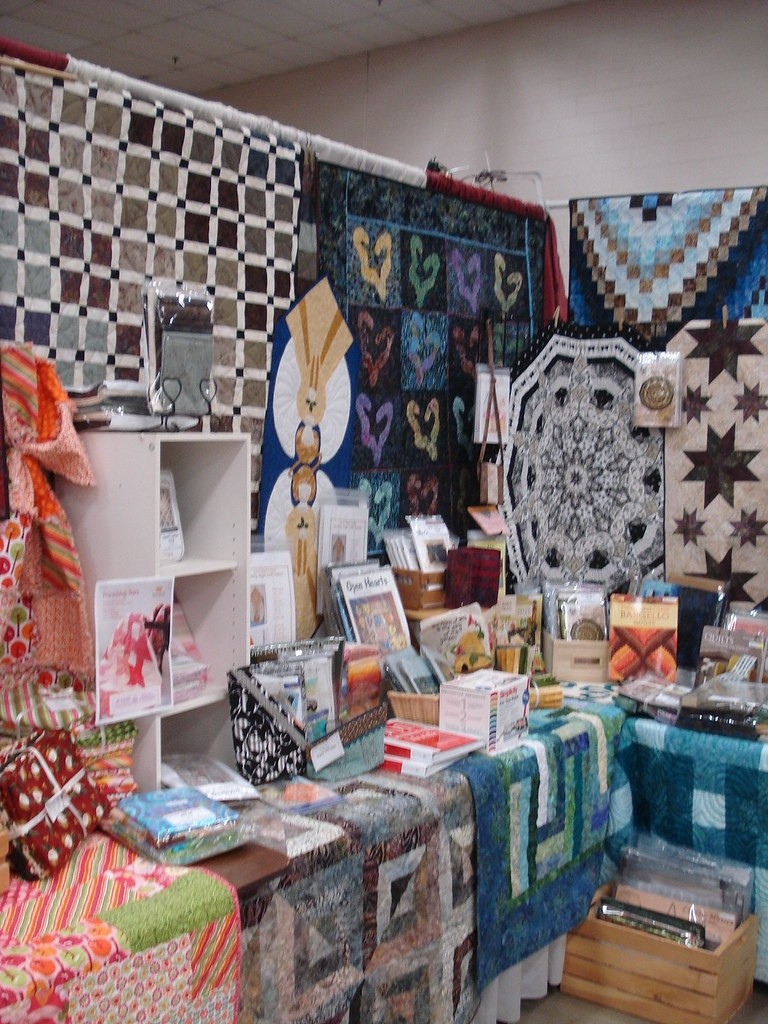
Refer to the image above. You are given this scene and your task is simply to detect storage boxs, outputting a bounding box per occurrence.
[439,670,530,752]
[541,631,610,682]
[561,883,758,1024]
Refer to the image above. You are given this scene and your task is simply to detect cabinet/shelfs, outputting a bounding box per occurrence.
[54,433,251,796]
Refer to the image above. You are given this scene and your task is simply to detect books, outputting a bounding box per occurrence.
[378,668,528,778]
[110,786,254,866]
[323,513,767,696]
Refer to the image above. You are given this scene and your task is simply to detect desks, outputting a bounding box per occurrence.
[0,686,768,1024]
